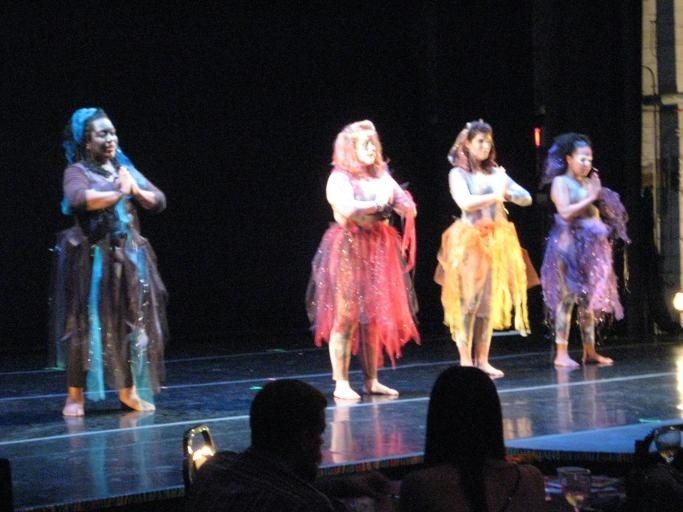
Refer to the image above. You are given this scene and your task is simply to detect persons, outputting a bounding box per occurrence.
[435,119,533,377]
[48,107,165,417]
[305,120,416,400]
[399,364,545,510]
[538,131,633,367]
[185,380,404,511]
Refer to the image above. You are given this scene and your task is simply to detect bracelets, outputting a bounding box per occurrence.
[378,494,402,501]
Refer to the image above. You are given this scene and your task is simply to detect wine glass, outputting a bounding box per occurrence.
[556,466,593,511]
